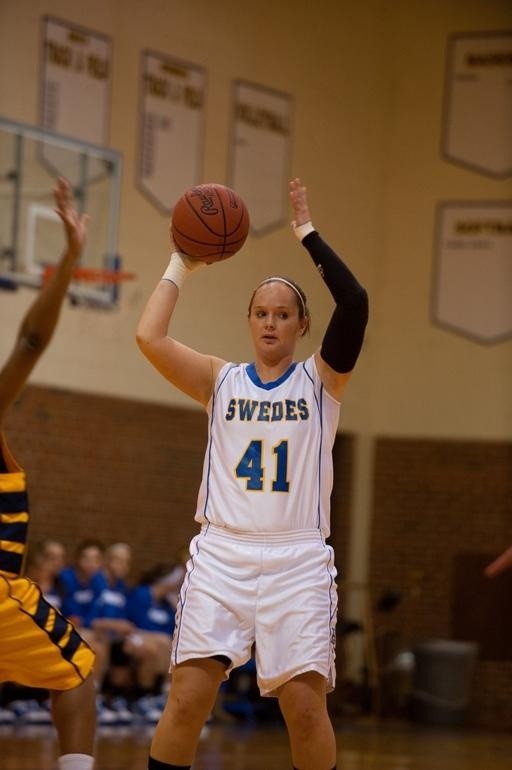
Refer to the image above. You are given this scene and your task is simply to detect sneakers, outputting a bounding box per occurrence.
[0,690,216,726]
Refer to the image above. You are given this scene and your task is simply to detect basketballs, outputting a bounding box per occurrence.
[170,183,251,266]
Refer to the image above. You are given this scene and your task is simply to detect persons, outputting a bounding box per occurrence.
[0,174,97,769]
[135,177,368,769]
